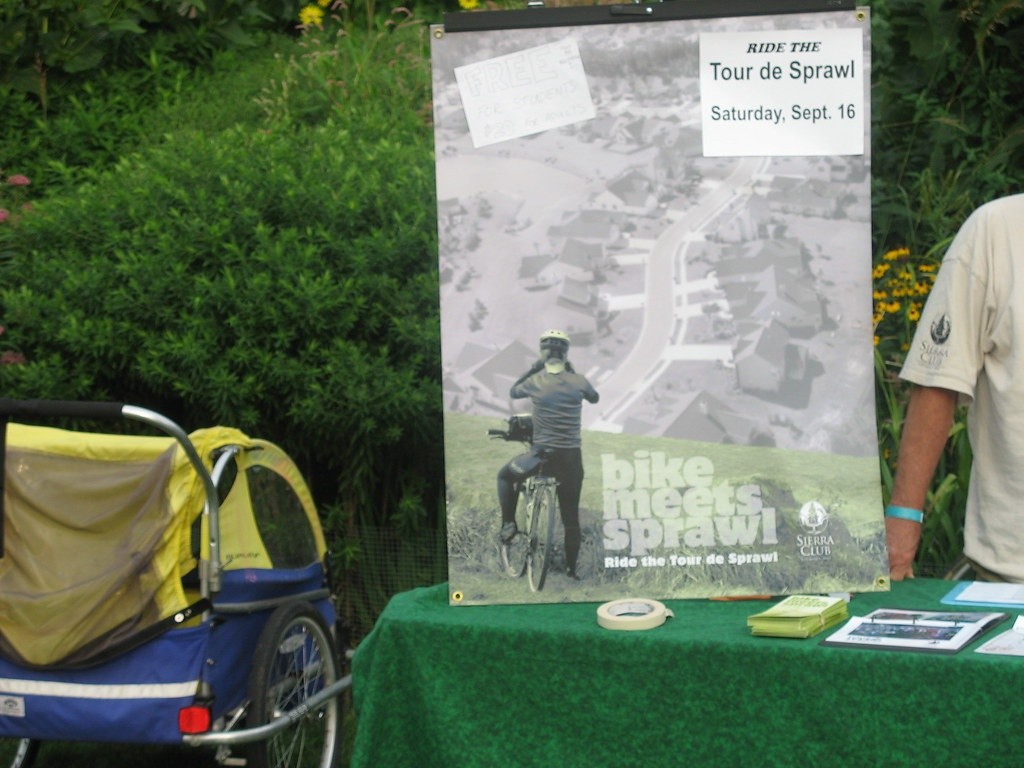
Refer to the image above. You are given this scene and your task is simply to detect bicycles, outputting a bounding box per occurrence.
[486,414,562,592]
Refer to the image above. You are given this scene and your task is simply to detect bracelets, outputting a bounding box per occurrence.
[884,502,929,531]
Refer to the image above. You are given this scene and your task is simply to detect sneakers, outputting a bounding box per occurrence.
[495,522,517,542]
[565,568,582,586]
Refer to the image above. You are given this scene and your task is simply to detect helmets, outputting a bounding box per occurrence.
[538,329,572,372]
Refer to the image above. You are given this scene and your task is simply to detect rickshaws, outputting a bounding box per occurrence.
[0,399,353,768]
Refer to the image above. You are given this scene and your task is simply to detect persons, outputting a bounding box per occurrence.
[491,329,600,582]
[878,189,1023,589]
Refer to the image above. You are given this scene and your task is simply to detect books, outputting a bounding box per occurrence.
[824,606,1013,655]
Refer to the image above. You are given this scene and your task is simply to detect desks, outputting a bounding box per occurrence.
[354,576,1023,766]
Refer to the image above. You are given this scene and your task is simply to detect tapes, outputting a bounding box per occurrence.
[595,598,675,631]
